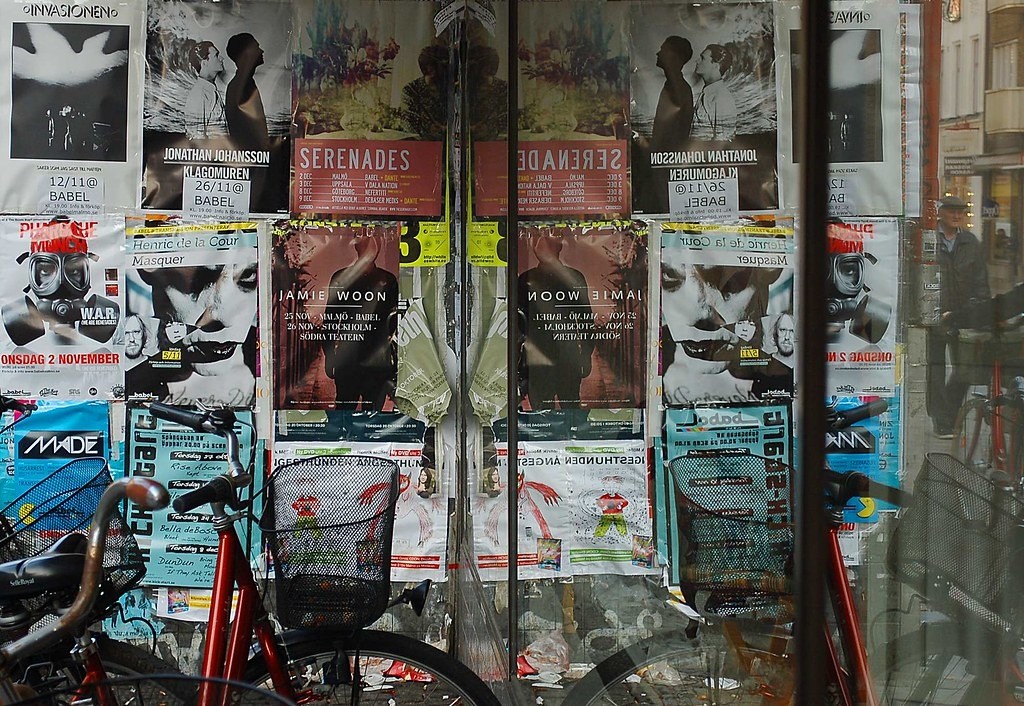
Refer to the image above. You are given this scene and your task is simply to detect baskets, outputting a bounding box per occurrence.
[668,452,796,627]
[0,457,146,626]
[257,455,400,631]
[886,452,1024,638]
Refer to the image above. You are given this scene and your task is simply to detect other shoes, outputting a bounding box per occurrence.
[932,423,956,438]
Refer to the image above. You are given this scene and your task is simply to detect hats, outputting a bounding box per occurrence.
[936,196,966,209]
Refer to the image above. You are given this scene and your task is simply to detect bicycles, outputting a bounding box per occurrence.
[559,400,916,705]
[942,312,1024,535]
[1,404,498,704]
[884,450,1024,706]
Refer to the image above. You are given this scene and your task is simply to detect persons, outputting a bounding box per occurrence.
[821,218,867,342]
[997,228,1015,251]
[28,214,91,301]
[920,196,990,442]
[513,226,597,408]
[682,43,741,176]
[658,262,795,406]
[635,36,694,194]
[417,468,433,496]
[321,223,402,411]
[125,215,260,411]
[403,41,447,143]
[486,467,500,493]
[184,40,241,166]
[461,43,509,143]
[222,32,277,172]
